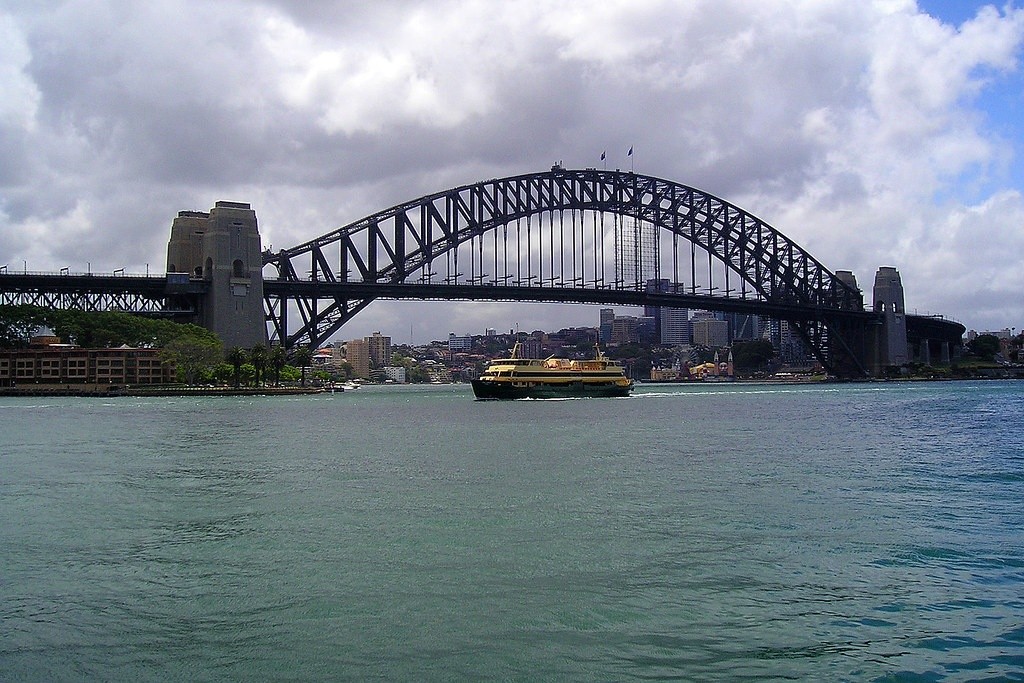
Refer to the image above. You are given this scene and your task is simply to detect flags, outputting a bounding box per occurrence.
[628,145,633,156]
[601,150,606,161]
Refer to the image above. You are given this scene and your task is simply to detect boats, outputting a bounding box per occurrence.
[470,340,635,400]
